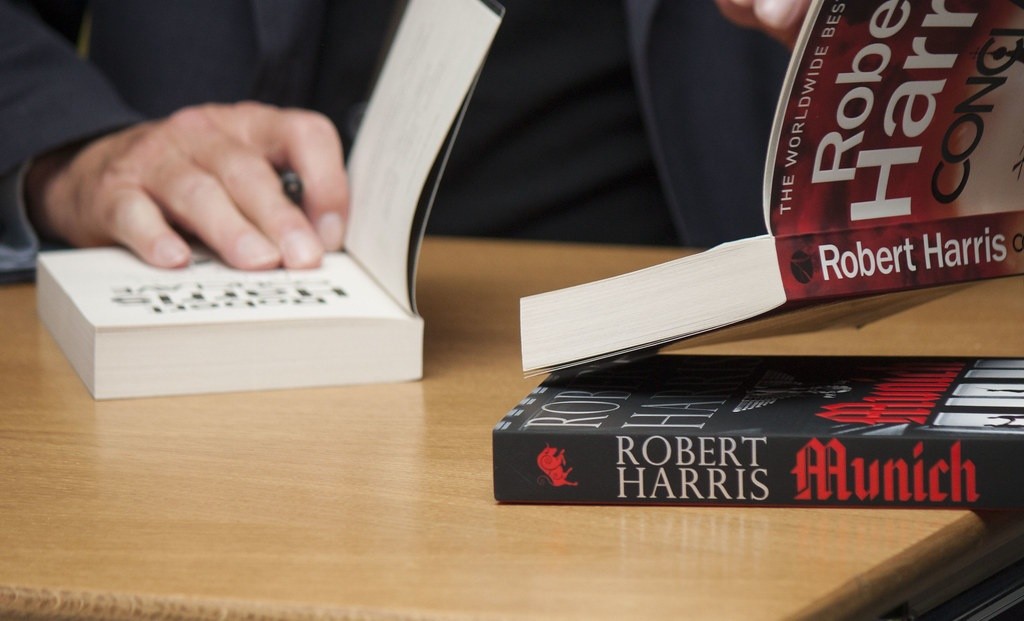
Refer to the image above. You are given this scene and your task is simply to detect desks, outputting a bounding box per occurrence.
[0,235,1024,620]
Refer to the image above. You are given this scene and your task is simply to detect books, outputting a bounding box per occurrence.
[37,0,511,404]
[517,0,1023,377]
[491,353,1023,511]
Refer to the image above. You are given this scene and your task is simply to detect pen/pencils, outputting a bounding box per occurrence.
[282,171,304,201]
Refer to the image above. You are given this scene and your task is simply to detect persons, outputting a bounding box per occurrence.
[0,0,809,271]
